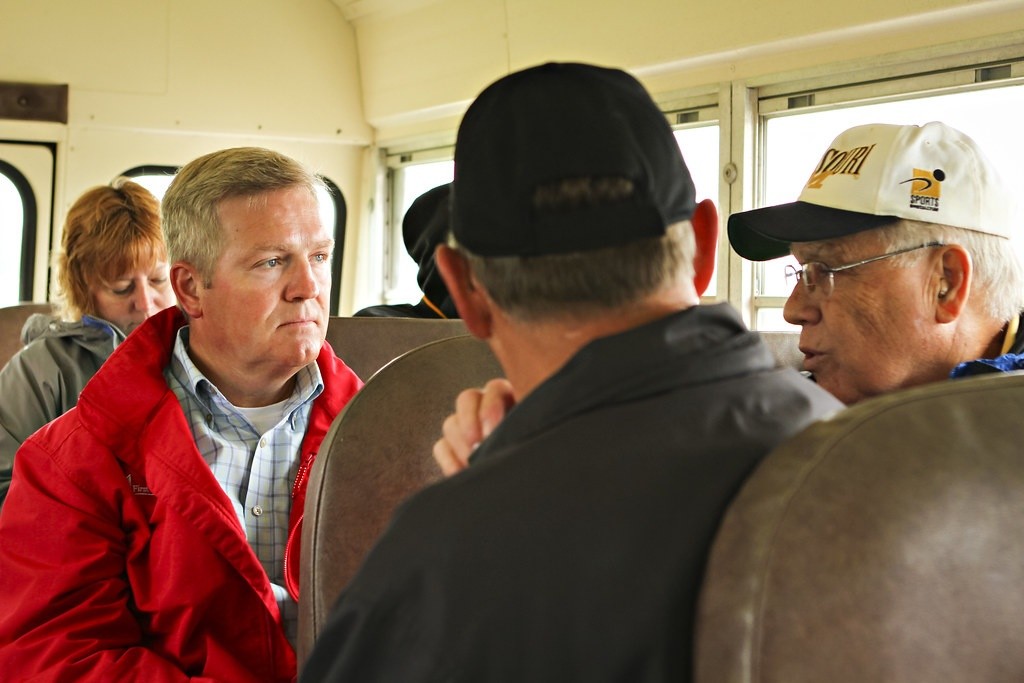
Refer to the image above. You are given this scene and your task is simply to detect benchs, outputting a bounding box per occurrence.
[295,316,1024,683]
[0,304,62,372]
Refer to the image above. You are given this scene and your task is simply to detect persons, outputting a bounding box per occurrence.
[0,147,369,683]
[293,63,853,682]
[728,120,1024,405]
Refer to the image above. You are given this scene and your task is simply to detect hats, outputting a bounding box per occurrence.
[452,63,697,257]
[728,122,1009,262]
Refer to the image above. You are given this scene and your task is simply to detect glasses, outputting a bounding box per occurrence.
[784,243,949,301]
